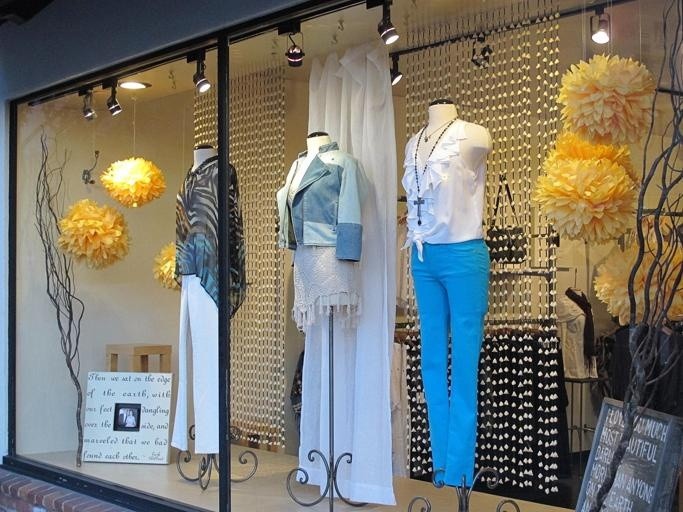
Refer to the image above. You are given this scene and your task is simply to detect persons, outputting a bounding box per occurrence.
[125,410,135,428]
[401,98,493,486]
[557,288,600,384]
[118,409,125,426]
[170,141,246,456]
[276,132,364,335]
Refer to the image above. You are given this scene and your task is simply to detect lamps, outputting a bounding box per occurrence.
[376,4,399,48]
[391,55,401,87]
[191,64,211,94]
[107,89,123,118]
[82,101,97,124]
[591,13,610,47]
[284,30,307,71]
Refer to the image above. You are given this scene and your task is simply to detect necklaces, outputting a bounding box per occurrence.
[185,154,221,192]
[423,121,450,143]
[413,117,455,225]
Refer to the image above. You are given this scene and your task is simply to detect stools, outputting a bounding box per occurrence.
[104,342,172,374]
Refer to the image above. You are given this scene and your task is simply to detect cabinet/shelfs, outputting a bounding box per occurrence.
[395,243,569,348]
